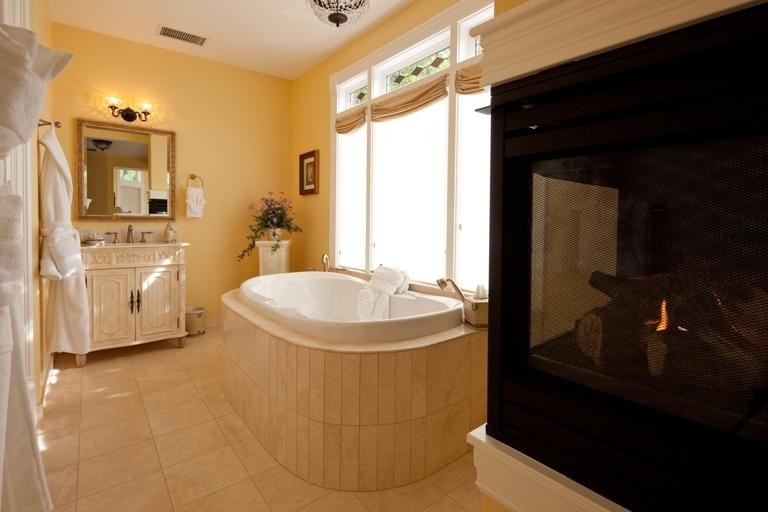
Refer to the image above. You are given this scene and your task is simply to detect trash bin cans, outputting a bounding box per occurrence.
[185,308,206,335]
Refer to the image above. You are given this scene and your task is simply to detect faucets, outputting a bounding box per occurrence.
[436,278,465,300]
[127,225,134,242]
[320,254,329,272]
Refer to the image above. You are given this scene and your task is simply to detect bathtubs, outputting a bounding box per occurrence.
[239,272,463,342]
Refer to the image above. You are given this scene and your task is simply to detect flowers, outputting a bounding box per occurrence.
[236,190,305,263]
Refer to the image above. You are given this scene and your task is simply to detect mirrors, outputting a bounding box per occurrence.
[76,118,176,222]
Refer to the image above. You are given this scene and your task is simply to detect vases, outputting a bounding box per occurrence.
[268,227,282,241]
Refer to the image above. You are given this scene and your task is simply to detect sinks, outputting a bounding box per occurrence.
[105,243,145,246]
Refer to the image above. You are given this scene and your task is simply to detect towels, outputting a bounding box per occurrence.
[357,265,411,322]
[185,186,207,219]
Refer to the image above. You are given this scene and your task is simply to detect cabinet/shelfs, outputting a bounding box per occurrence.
[85,246,179,348]
[254,239,291,275]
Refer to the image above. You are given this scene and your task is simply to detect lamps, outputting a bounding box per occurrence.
[307,0,369,27]
[108,96,151,121]
[92,139,112,152]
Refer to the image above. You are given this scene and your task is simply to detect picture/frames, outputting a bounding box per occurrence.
[298,149,319,195]
